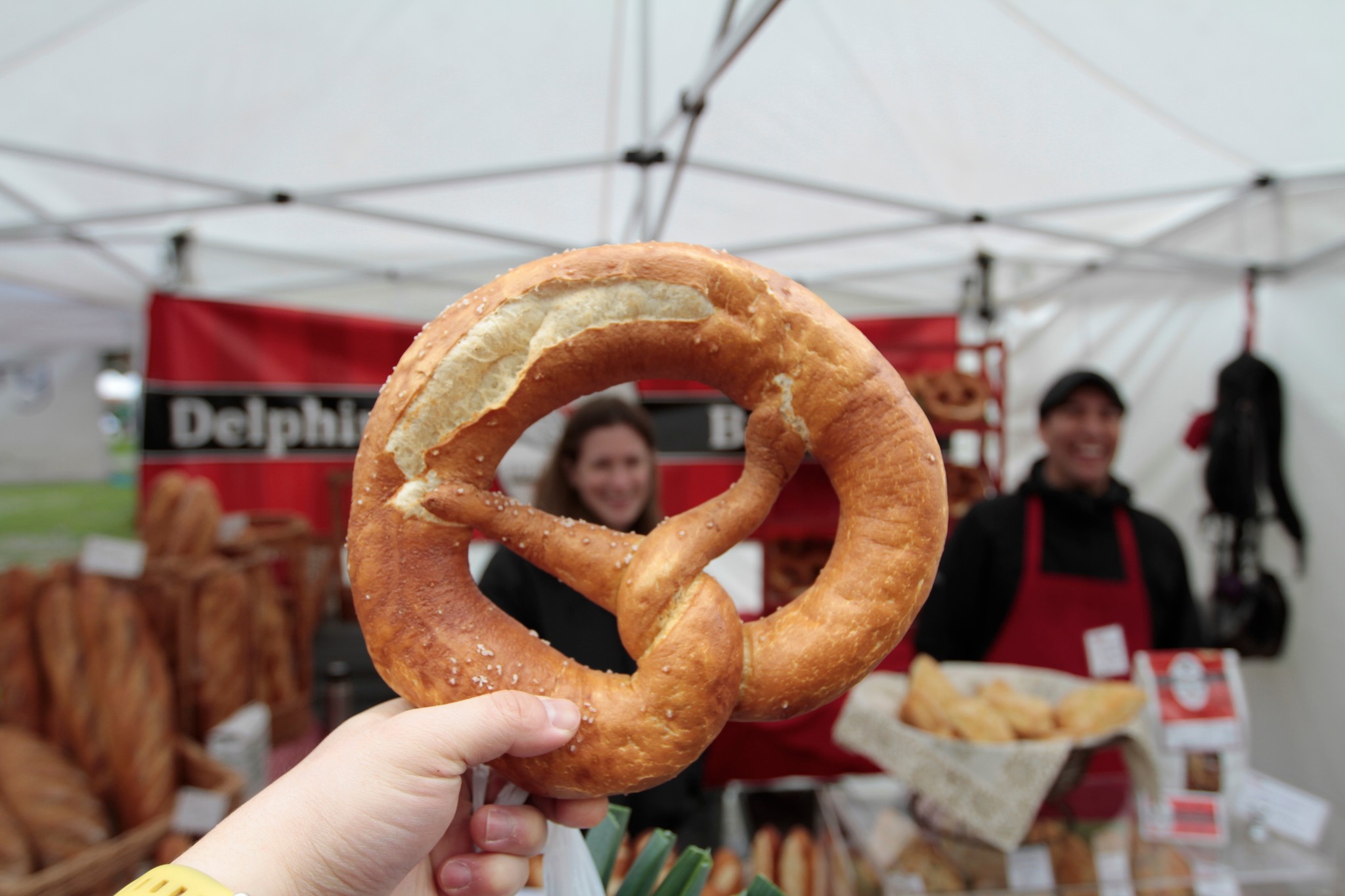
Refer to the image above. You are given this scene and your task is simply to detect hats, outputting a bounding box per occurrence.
[1039,370,1123,418]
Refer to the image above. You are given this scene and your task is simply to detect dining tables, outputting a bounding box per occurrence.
[1195,808,1337,896]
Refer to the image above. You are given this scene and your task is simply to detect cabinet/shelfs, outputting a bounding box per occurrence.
[734,340,1009,625]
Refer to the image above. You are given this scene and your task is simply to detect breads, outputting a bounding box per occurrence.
[344,240,947,803]
[0,466,340,896]
[602,650,1204,896]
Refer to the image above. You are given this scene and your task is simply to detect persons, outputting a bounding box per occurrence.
[101,684,616,893]
[465,396,721,858]
[913,367,1210,689]
